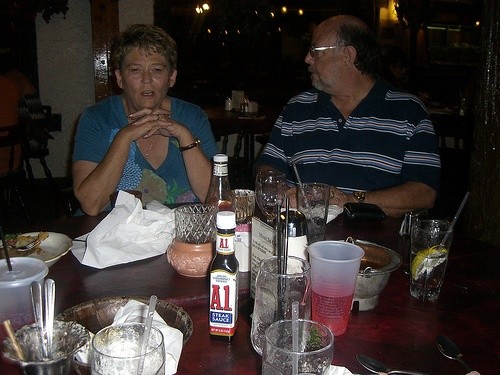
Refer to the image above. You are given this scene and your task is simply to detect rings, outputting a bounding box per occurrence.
[158,114,160,120]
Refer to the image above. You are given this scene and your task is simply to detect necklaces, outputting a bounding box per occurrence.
[126,105,158,157]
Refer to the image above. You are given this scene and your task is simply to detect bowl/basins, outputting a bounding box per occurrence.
[55,296,194,374]
[0,232,74,268]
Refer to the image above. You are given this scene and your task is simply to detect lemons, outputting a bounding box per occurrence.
[410,246,447,280]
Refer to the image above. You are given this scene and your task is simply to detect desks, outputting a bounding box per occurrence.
[0,203,500,375]
[203,105,280,129]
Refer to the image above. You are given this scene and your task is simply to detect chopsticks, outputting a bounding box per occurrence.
[277,197,290,319]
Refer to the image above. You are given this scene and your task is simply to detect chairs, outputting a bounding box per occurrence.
[0,106,55,224]
[435,114,475,198]
[213,125,273,190]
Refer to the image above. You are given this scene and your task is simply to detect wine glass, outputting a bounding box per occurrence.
[255,169,286,225]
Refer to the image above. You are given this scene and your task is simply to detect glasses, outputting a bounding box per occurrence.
[308,44,360,58]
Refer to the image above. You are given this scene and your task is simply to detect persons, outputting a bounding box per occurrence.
[254,15,441,215]
[73,24,220,216]
[0,73,37,173]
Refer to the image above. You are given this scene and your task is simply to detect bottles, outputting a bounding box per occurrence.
[208,211,240,342]
[165,239,213,278]
[4,319,91,375]
[240,100,249,116]
[203,154,234,207]
[248,207,309,309]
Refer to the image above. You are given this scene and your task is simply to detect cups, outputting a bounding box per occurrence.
[251,255,311,356]
[296,182,330,240]
[0,257,49,364]
[306,242,365,337]
[175,204,219,244]
[409,220,454,302]
[92,323,165,375]
[262,320,334,375]
[230,189,255,222]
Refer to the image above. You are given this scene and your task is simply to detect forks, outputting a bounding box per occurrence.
[0,235,49,252]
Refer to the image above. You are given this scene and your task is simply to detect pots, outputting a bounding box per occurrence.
[335,237,402,312]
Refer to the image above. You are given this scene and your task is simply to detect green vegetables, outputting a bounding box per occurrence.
[4,233,21,242]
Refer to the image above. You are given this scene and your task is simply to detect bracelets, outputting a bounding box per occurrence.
[179,138,200,151]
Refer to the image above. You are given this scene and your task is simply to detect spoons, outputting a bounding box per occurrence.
[356,354,427,375]
[435,333,472,373]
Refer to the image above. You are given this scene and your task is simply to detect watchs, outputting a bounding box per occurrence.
[353,190,365,203]
